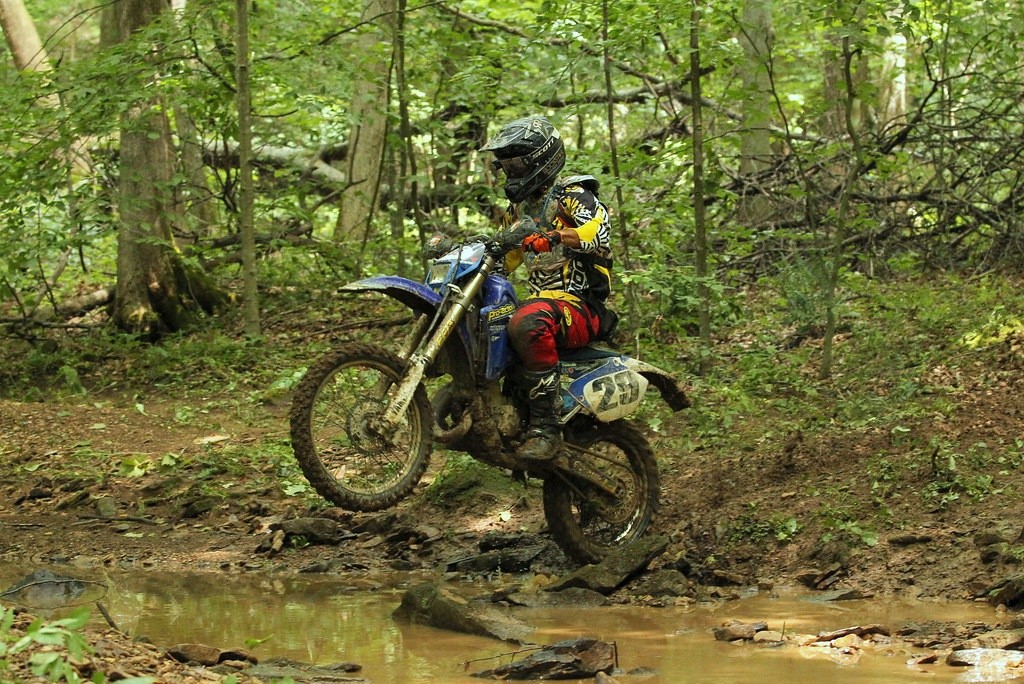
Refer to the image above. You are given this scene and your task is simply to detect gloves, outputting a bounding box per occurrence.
[522,232,562,255]
[422,229,452,259]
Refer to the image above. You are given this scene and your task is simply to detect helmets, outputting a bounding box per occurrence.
[478,117,567,203]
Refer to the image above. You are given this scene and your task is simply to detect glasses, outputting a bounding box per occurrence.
[499,127,559,179]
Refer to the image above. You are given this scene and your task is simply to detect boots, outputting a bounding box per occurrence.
[516,370,562,461]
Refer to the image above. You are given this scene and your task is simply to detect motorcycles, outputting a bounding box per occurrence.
[290,216,694,568]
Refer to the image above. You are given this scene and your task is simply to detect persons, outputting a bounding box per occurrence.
[481,113,618,465]
